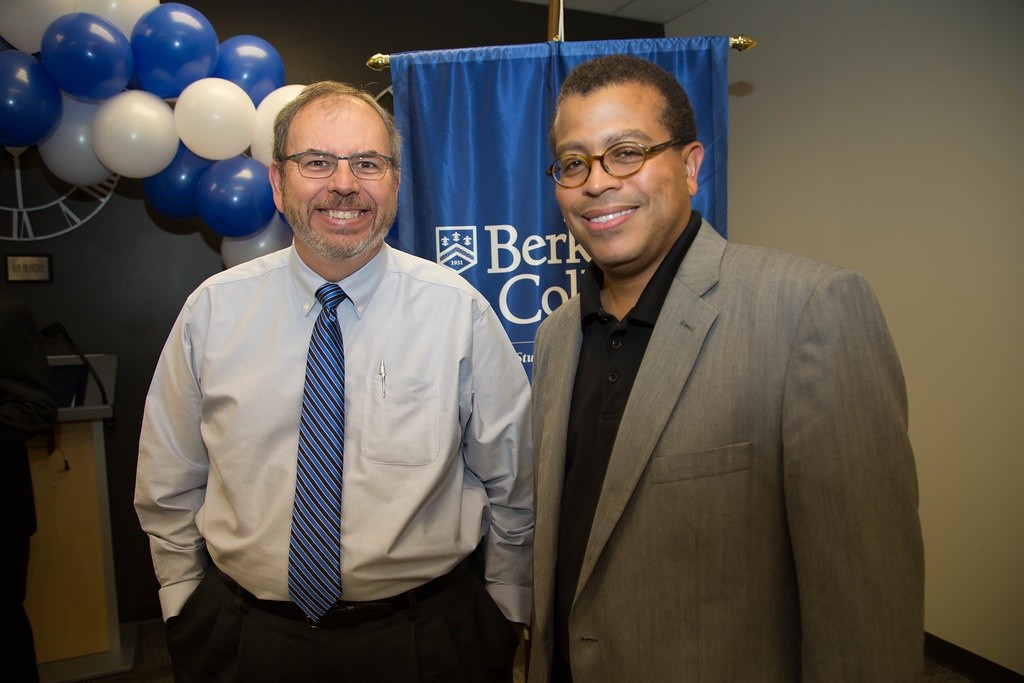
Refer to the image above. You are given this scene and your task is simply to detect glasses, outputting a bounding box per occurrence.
[545,136,688,188]
[281,150,395,180]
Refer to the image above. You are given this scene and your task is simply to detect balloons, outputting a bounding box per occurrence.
[128,2,222,98]
[172,75,257,162]
[197,156,275,238]
[38,88,115,188]
[221,208,293,272]
[144,138,214,221]
[214,32,288,106]
[1,46,64,148]
[92,88,181,179]
[251,83,313,170]
[39,12,135,102]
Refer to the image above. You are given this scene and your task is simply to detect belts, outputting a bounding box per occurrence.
[202,545,486,631]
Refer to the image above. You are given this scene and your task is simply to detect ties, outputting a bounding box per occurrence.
[286,280,345,625]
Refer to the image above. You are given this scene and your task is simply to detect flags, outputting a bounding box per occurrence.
[387,34,729,389]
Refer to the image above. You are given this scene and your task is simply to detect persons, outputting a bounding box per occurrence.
[131,82,535,683]
[517,56,924,683]
